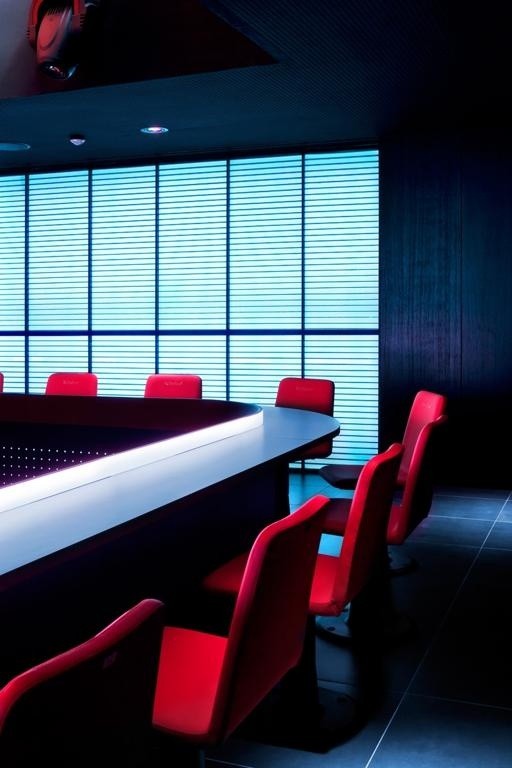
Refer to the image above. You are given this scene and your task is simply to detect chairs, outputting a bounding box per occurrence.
[207,449,400,688]
[324,388,447,492]
[44,373,100,396]
[314,414,448,537]
[1,590,166,767]
[275,375,342,463]
[142,374,206,399]
[153,494,328,752]
